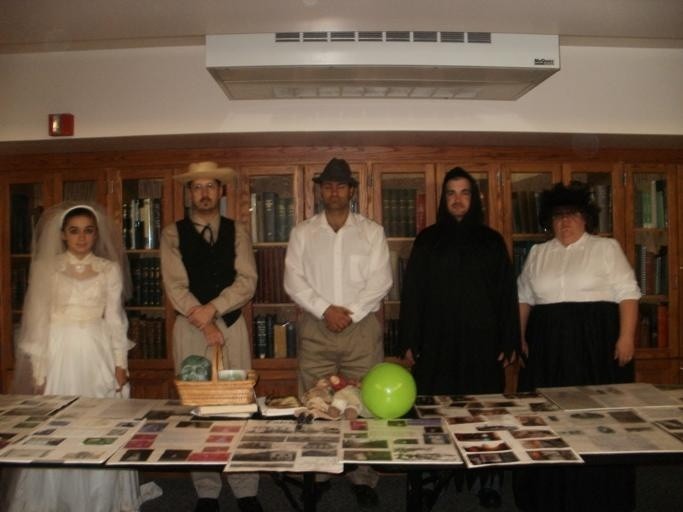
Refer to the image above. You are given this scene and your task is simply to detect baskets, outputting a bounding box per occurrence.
[174,344,259,406]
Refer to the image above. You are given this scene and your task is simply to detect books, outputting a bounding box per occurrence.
[589,179,670,351]
[478,184,553,280]
[314,188,426,357]
[184,192,297,358]
[13,192,166,359]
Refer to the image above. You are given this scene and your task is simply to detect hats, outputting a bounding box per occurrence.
[172,160,239,186]
[312,157,359,187]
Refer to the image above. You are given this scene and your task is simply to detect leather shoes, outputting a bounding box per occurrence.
[237,496,264,512]
[299,479,332,507]
[353,484,379,510]
[478,488,501,510]
[196,498,220,512]
[406,488,434,511]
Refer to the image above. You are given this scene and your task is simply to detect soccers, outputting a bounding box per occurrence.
[361,363,417,419]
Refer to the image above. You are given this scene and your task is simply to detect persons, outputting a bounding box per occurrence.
[292,359,418,435]
[157,158,265,509]
[18,203,135,512]
[511,179,643,509]
[282,158,399,509]
[396,164,525,511]
